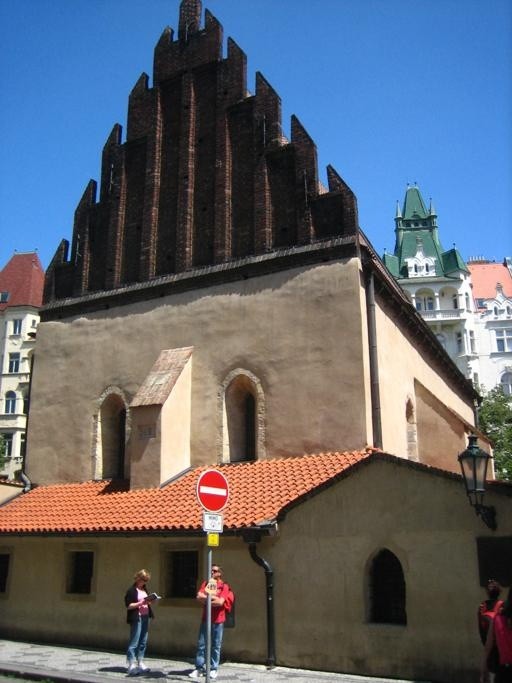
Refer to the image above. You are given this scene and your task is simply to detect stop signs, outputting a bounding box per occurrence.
[196,469,229,512]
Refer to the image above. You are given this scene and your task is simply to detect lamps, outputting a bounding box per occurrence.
[457,434,495,529]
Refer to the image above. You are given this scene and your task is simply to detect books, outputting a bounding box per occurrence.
[143,591,162,603]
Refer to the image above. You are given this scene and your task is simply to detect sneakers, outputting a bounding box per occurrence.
[188,669,204,678]
[210,669,217,679]
[128,665,150,676]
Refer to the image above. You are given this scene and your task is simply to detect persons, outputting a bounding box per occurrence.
[124,567,157,676]
[187,563,234,679]
[481,583,511,683]
[477,581,505,683]
